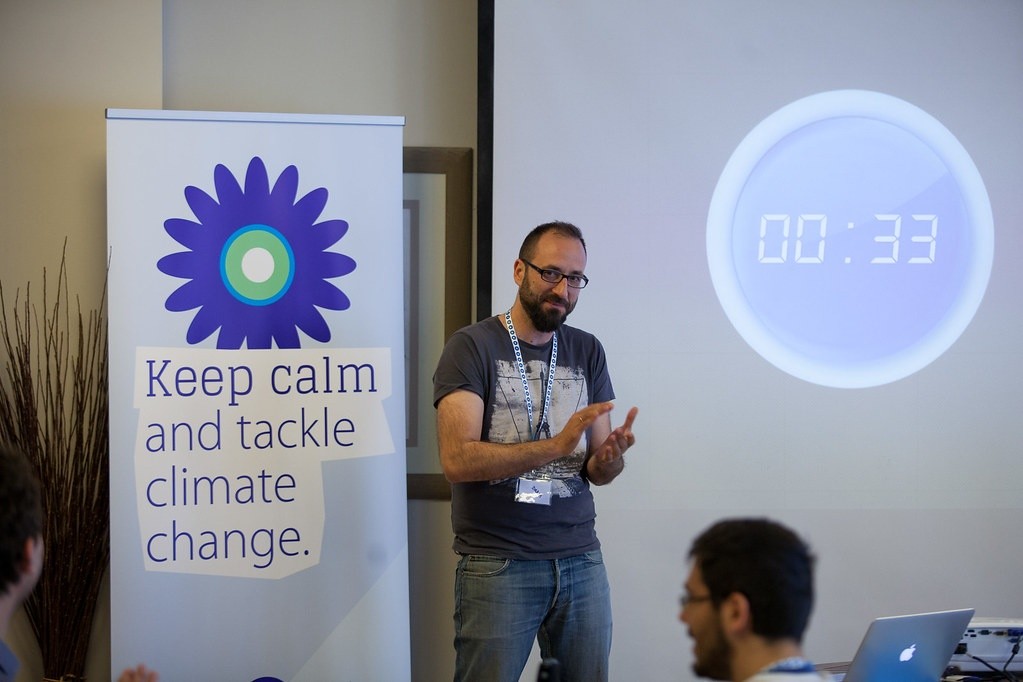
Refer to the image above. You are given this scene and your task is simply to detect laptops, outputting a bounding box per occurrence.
[841,608,975,682]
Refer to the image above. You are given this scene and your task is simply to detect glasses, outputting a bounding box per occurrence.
[681,589,733,612]
[521,258,589,289]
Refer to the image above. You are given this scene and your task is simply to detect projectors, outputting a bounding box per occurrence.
[947,618,1023,672]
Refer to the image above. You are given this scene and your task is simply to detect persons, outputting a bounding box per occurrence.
[433,221,639,682]
[680,517,826,682]
[0,448,158,682]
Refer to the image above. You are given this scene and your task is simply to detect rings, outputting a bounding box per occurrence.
[580,417,584,422]
